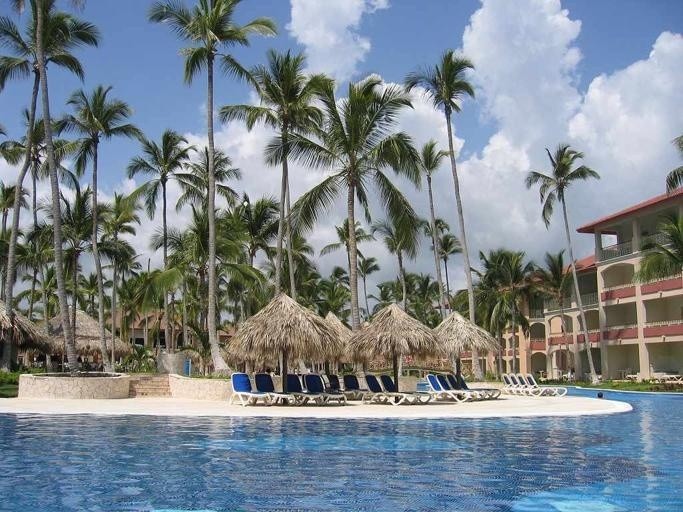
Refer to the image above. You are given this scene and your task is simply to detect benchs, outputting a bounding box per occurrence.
[626,374,683,384]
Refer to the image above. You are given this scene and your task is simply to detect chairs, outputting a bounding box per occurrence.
[364,374,416,407]
[33,360,138,372]
[343,376,368,400]
[304,373,348,406]
[322,374,356,401]
[499,372,568,398]
[229,372,268,406]
[286,372,322,406]
[255,373,295,405]
[425,373,501,404]
[380,374,432,406]
